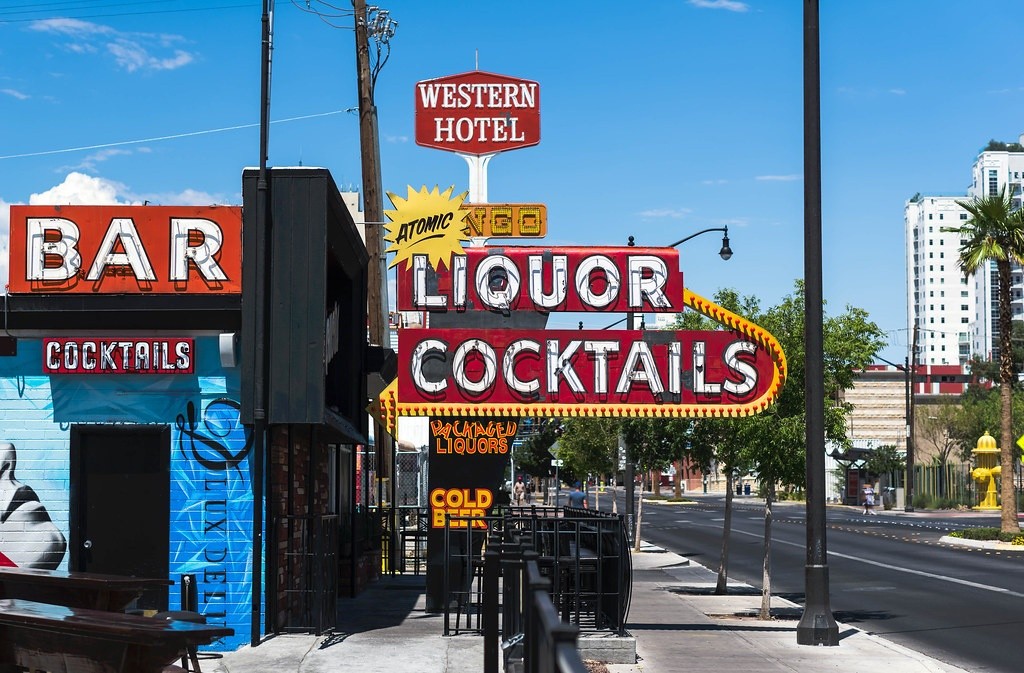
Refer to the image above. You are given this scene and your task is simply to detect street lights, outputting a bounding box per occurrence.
[623,225,734,548]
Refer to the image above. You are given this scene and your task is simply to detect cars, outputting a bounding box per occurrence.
[502,479,562,495]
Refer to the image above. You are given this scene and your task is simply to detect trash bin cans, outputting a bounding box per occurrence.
[736,484,742,495]
[744,485,751,494]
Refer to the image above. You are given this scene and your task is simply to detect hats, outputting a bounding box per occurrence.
[518,476,522,481]
[574,482,581,487]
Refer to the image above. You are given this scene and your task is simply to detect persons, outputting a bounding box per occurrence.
[862,482,877,515]
[514,476,525,506]
[568,481,588,509]
[525,479,532,504]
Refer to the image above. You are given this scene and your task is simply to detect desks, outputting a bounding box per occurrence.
[0,567,236,673]
